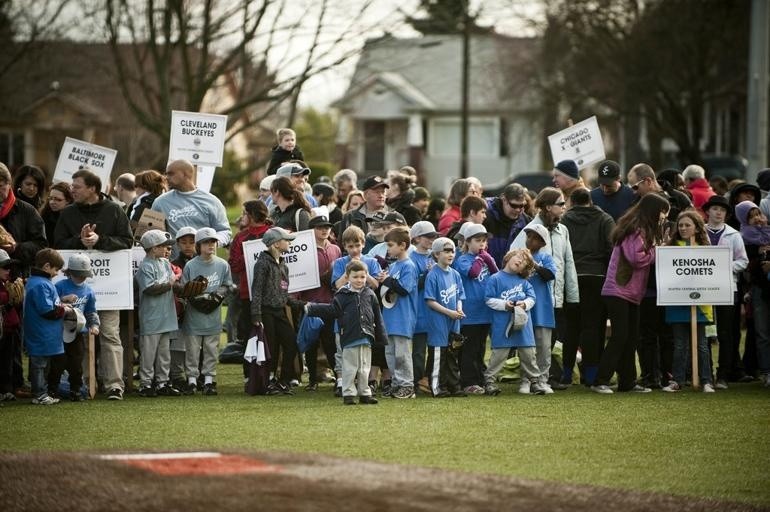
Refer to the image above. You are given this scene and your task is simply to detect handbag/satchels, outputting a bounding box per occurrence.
[243,322,271,397]
[442,311,471,359]
[499,348,524,382]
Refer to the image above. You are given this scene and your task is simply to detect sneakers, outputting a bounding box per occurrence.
[1,378,218,404]
[359,394,378,405]
[343,395,356,406]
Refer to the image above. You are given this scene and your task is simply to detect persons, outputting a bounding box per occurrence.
[550,160,770,394]
[1,161,233,404]
[304,261,389,404]
[267,128,305,175]
[229,163,580,397]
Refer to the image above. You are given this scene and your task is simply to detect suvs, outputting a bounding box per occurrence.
[675,155,749,182]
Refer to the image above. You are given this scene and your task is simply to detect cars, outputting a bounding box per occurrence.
[485,171,557,197]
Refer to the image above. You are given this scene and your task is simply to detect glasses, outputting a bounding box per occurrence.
[631,180,645,190]
[507,199,524,208]
[555,201,565,206]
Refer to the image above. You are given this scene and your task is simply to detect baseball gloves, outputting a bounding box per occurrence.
[178,275,208,298]
[187,293,225,314]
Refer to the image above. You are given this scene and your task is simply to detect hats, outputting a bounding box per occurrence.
[364,210,489,252]
[520,222,553,247]
[504,304,529,339]
[730,183,761,206]
[275,163,311,179]
[702,195,732,214]
[378,278,399,310]
[597,159,621,184]
[67,251,95,279]
[0,244,24,270]
[61,306,88,347]
[138,226,219,250]
[362,175,389,190]
[553,158,580,181]
[260,225,296,247]
[307,216,334,228]
